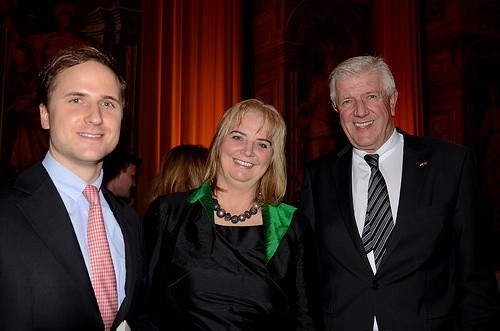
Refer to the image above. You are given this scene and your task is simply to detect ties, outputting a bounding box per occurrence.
[361,153,395,271]
[82,184,118,331]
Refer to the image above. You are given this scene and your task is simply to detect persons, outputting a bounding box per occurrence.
[296,56,483,331]
[101,150,138,206]
[1,44,144,331]
[147,144,220,205]
[138,100,314,331]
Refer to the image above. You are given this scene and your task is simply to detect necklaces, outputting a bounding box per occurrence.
[211,190,266,222]
